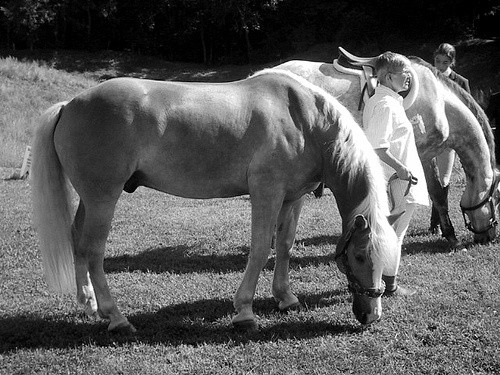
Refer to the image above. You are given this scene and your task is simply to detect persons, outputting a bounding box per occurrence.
[363,52,430,296]
[429,42,470,234]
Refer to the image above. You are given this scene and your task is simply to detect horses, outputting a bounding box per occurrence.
[32,67,406,337]
[271,55,500,254]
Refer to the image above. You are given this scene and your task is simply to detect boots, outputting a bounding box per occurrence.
[429,185,450,234]
